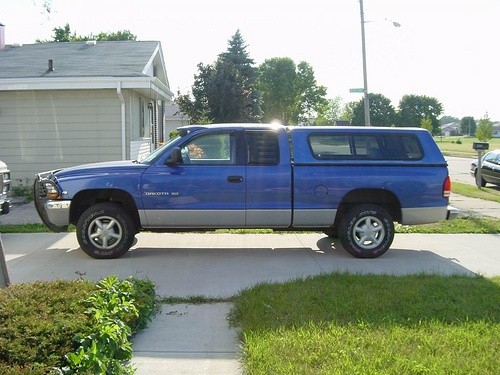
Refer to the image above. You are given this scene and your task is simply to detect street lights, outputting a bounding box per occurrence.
[359,16,404,30]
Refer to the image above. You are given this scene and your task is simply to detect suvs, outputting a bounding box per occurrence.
[30,120,460,261]
[0,159,14,219]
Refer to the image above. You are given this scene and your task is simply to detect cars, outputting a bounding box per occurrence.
[470,148,500,189]
